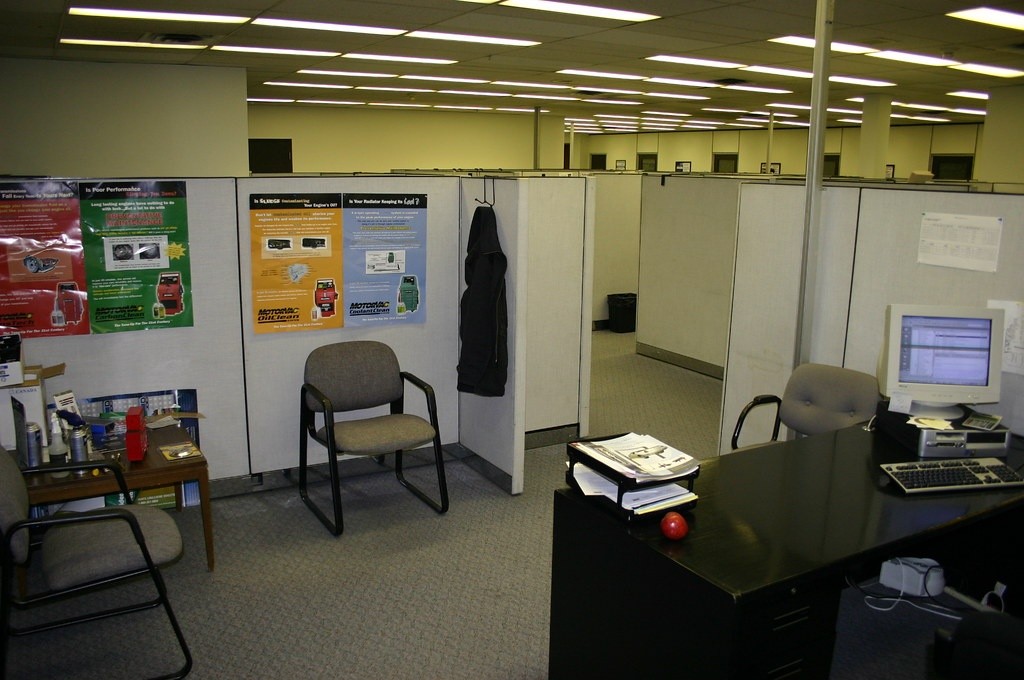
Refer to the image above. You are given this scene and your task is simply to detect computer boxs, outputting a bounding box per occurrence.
[873,401,1010,465]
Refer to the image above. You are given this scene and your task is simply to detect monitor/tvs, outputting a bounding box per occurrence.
[875,303,1004,421]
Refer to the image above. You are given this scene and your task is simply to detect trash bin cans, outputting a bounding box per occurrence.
[607,293,637,333]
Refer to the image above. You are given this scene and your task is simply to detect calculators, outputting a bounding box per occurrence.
[962,411,1003,431]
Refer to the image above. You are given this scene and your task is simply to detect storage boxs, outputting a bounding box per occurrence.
[0,332,82,451]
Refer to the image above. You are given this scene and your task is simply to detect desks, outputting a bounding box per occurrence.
[549,420,1024,680]
[6,418,214,596]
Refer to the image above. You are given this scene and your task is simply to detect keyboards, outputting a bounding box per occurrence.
[880,457,1024,495]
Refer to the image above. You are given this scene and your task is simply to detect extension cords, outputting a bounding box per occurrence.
[944,587,998,612]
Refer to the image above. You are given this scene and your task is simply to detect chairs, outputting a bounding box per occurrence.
[933,613,1023,680]
[299,341,449,535]
[0,444,193,680]
[731,364,880,453]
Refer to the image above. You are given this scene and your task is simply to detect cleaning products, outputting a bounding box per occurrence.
[49,412,69,479]
